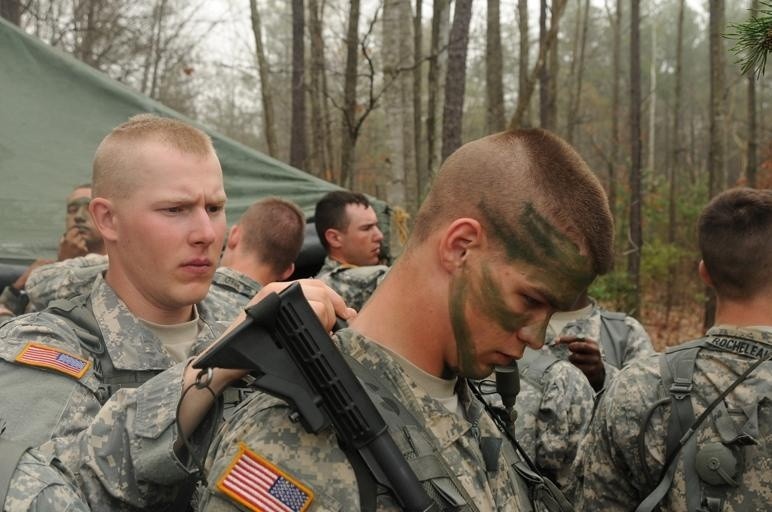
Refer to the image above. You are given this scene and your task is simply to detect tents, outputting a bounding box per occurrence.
[1,17,410,285]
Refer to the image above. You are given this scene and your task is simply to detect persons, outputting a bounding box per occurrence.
[192,127,613,512]
[0,257,55,324]
[24,194,307,324]
[544,286,653,394]
[316,189,389,314]
[56,184,110,263]
[471,348,596,487]
[0,113,360,512]
[565,187,772,512]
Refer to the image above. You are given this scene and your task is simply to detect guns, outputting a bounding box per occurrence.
[192,282,441,512]
